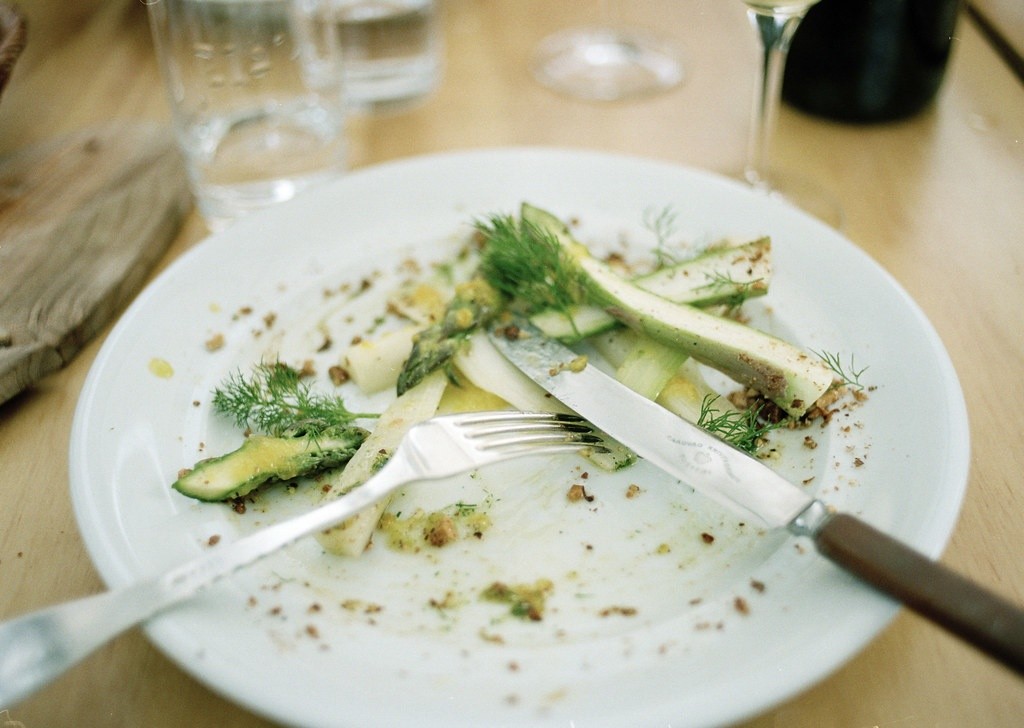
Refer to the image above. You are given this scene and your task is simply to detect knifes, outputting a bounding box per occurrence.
[486,308,1024,691]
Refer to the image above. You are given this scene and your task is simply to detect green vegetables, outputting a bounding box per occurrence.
[209,209,869,453]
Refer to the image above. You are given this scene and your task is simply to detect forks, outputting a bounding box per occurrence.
[0,405,609,724]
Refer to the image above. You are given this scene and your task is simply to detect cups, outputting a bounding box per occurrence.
[288,3,438,109]
[144,1,362,239]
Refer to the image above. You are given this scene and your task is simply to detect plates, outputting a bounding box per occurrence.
[67,149,971,728]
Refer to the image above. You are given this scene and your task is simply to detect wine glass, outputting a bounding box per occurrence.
[680,0,845,238]
